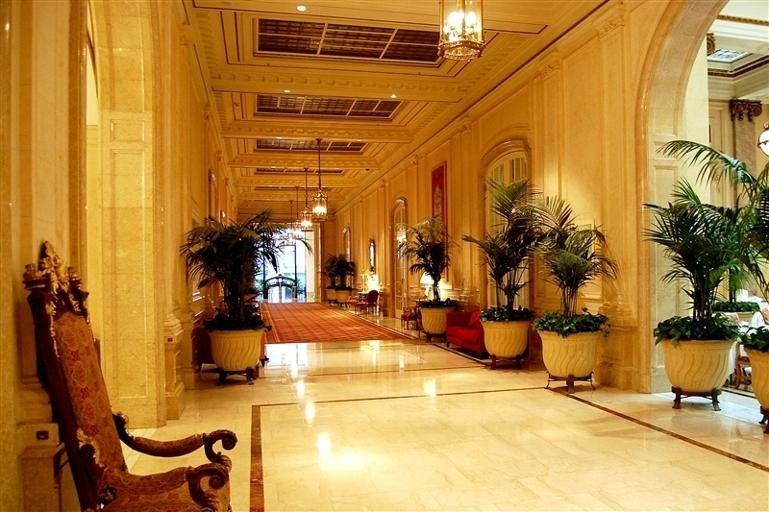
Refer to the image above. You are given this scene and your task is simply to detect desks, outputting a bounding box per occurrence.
[358,292,369,311]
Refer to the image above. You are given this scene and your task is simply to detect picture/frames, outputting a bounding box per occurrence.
[431,159,450,282]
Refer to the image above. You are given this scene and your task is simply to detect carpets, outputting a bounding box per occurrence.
[258,301,415,344]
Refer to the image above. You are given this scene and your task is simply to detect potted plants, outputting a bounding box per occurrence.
[396,214,458,343]
[642,175,741,412]
[655,135,769,437]
[331,253,354,307]
[529,194,619,394]
[460,176,546,370]
[710,201,760,357]
[316,253,339,306]
[178,208,280,385]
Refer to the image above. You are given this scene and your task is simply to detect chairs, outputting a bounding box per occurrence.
[404,303,419,330]
[400,306,411,326]
[347,295,358,310]
[22,239,239,512]
[445,305,489,360]
[357,290,378,314]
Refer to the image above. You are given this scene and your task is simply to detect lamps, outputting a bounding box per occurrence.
[311,137,329,222]
[288,168,312,239]
[436,0,485,62]
